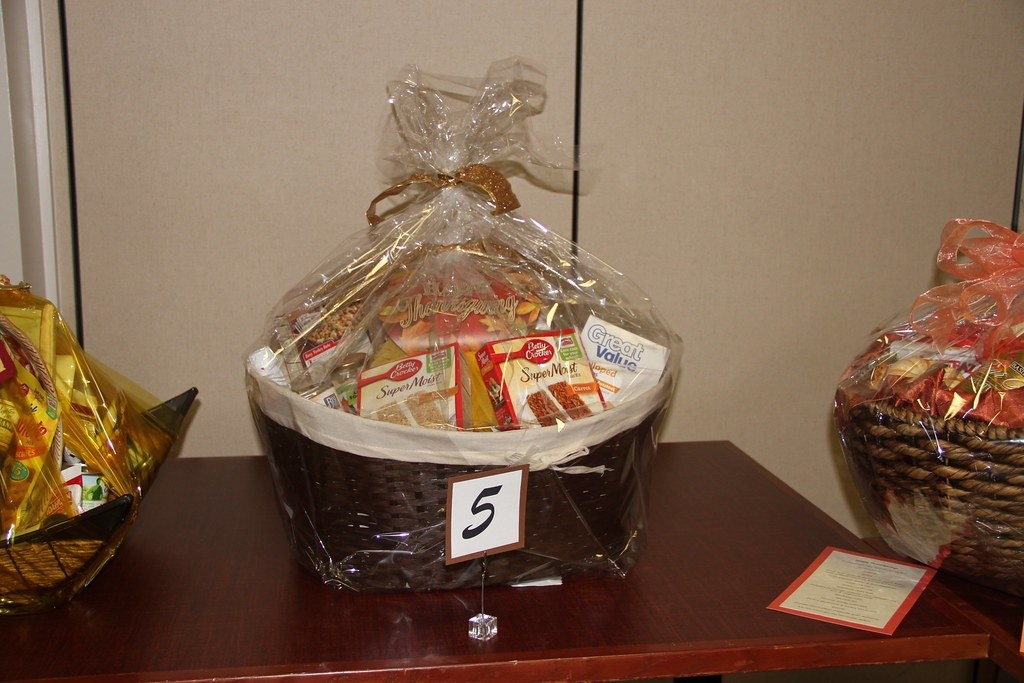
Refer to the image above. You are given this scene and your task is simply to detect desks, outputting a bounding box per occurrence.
[1,438,1024,683]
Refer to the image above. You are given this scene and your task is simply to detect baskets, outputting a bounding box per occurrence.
[836,382,1024,580]
[242,339,672,592]
[0,310,200,614]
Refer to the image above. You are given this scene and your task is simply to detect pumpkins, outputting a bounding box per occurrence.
[884,356,964,390]
